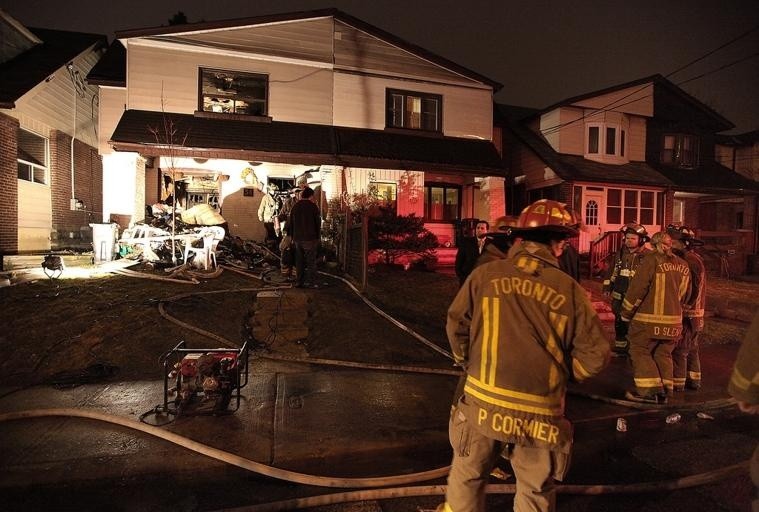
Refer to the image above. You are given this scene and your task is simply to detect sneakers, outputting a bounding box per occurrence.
[625,382,701,405]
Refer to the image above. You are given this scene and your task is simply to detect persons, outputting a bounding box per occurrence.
[277,186,304,284]
[668,224,706,393]
[726,315,758,415]
[454,219,489,290]
[619,230,696,405]
[436,197,614,512]
[257,182,284,251]
[286,187,323,290]
[475,214,520,270]
[601,222,652,356]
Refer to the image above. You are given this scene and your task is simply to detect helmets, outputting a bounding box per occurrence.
[516,202,529,232]
[621,223,650,242]
[668,225,704,246]
[500,199,583,238]
[478,217,520,240]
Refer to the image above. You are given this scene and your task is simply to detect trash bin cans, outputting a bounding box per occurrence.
[88,222,120,266]
